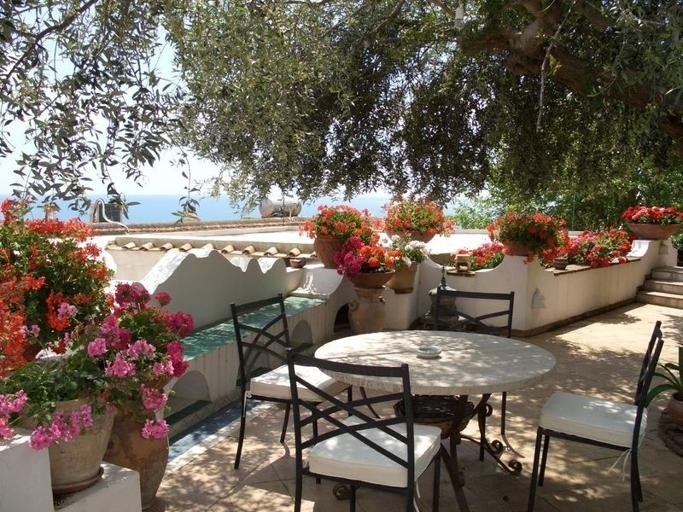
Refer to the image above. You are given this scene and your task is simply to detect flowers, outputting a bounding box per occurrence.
[298,190,456,274]
[0,196,198,449]
[455,206,682,269]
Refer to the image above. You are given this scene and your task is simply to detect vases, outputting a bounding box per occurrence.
[345,269,396,288]
[10,396,119,494]
[101,398,172,511]
[388,261,419,295]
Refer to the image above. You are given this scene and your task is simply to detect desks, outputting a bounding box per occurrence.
[310,322,560,499]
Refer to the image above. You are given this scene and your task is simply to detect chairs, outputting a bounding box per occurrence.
[284,348,445,512]
[226,292,355,486]
[423,283,520,464]
[526,316,665,511]
